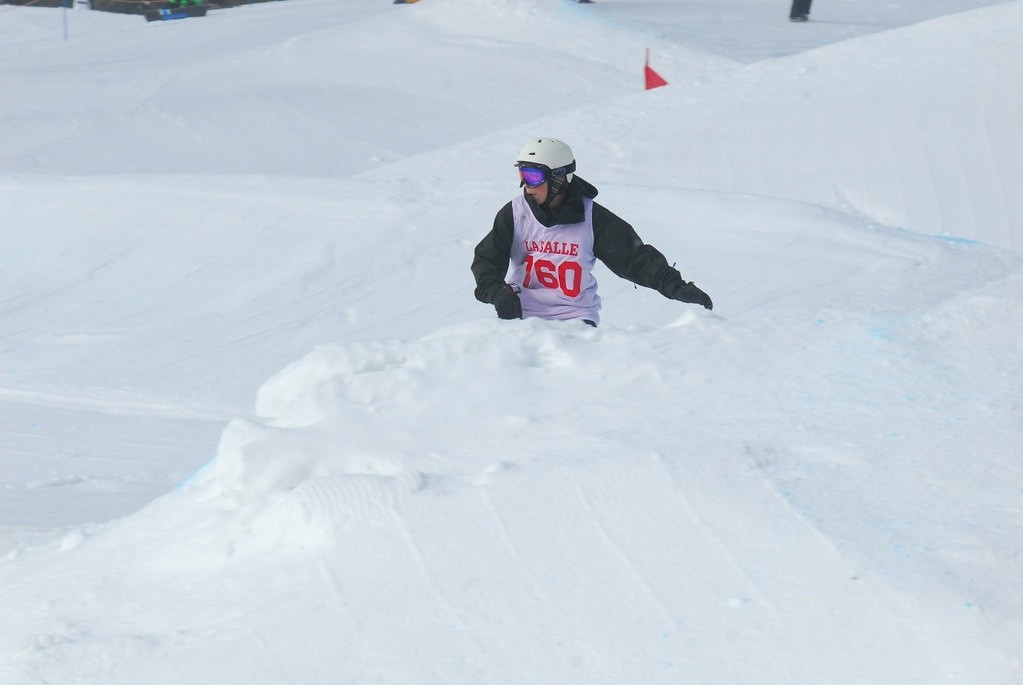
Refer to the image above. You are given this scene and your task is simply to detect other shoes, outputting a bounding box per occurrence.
[790,14,810,22]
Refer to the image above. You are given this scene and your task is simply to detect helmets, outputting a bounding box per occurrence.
[514,137,577,176]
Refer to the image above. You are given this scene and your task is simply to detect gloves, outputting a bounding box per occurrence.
[487,281,523,320]
[673,281,713,310]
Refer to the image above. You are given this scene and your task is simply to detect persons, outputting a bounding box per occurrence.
[470,138,714,331]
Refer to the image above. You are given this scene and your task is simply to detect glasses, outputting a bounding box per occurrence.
[519,166,545,188]
